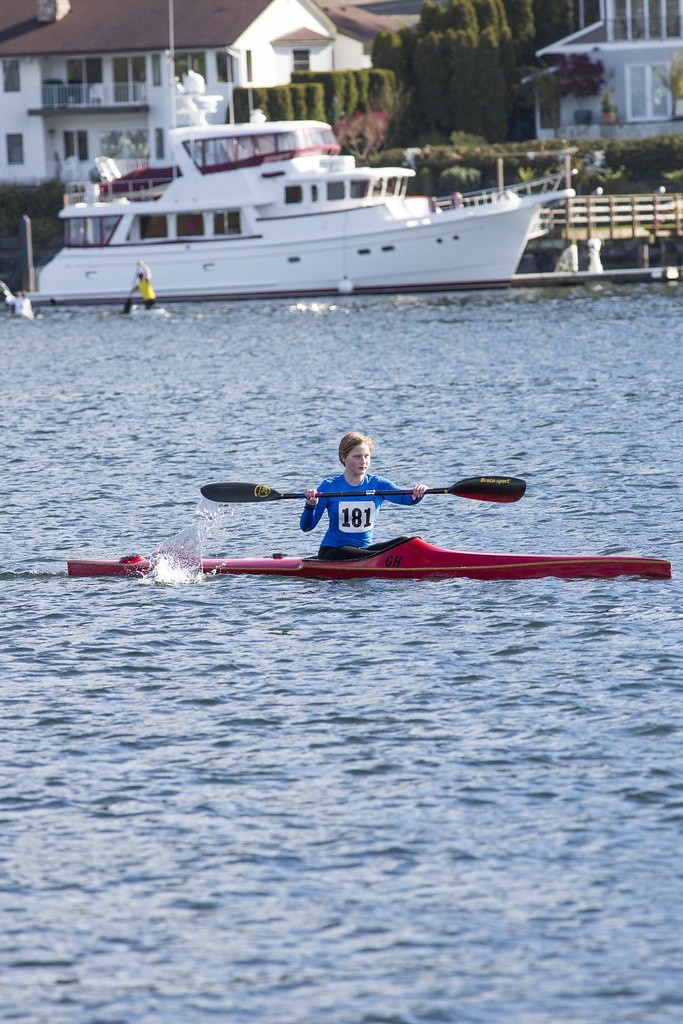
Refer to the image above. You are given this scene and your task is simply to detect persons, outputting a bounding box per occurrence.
[130,258,156,309]
[300,432,428,561]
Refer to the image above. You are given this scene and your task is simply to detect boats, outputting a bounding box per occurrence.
[65,535,675,580]
[7,0,578,323]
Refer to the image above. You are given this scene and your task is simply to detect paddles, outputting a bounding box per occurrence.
[123,263,139,315]
[200,475,526,503]
